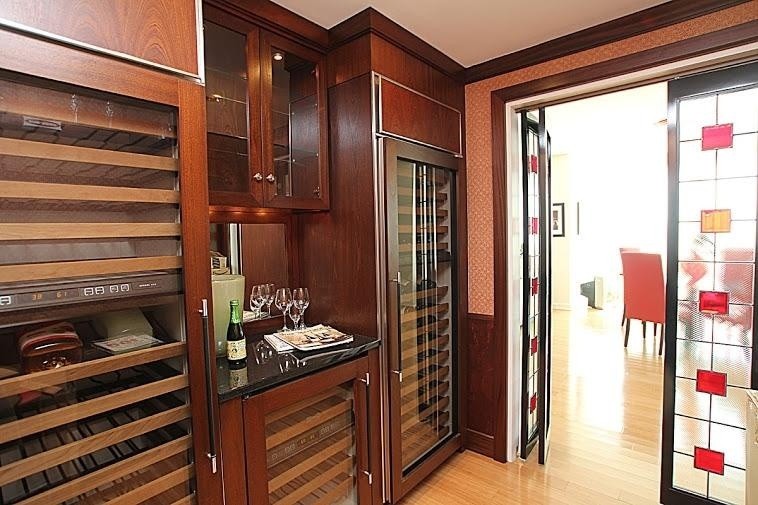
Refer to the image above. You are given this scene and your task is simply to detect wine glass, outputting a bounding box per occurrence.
[250,284,275,321]
[275,287,310,334]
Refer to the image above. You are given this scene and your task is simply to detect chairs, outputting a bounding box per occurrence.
[619,246,756,356]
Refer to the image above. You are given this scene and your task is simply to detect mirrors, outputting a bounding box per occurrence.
[208,222,292,344]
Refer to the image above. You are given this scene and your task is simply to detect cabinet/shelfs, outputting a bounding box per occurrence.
[1,0,216,504]
[201,0,331,214]
[299,32,470,505]
[215,338,382,505]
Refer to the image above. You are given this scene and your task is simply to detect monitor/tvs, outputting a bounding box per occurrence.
[581,277,605,310]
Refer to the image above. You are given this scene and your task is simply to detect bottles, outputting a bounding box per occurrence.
[226,300,247,365]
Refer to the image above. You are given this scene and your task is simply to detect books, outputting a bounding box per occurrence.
[272,325,355,351]
[263,332,296,353]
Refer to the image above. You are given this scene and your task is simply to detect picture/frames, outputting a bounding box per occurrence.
[551,202,566,237]
